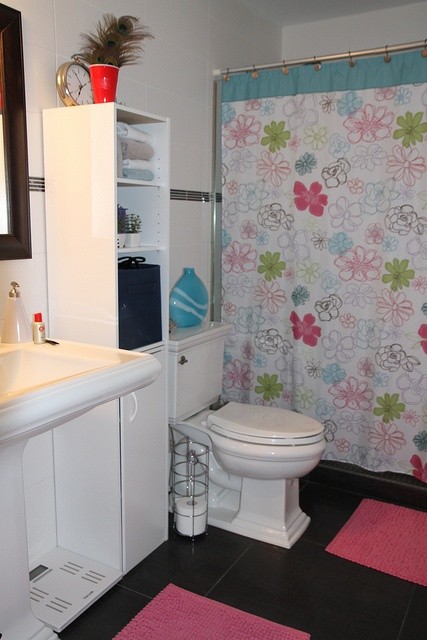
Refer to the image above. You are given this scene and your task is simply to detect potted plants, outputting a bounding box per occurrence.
[118,210,129,249]
[70,12,156,105]
[125,213,143,253]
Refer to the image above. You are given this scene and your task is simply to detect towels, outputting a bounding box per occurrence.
[123,158,155,170]
[117,121,152,143]
[123,169,154,181]
[121,138,155,161]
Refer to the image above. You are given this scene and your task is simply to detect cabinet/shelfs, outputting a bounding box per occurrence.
[40,101,172,573]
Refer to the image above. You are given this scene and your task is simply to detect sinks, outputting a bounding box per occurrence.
[0,345,150,407]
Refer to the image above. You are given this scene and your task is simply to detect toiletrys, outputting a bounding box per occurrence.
[31,312,47,345]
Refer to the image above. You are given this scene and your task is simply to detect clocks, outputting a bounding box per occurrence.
[56,56,94,107]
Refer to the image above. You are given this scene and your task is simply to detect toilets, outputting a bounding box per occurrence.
[169,322,330,549]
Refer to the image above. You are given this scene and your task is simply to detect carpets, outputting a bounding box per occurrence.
[323,498,426,591]
[112,581,310,640]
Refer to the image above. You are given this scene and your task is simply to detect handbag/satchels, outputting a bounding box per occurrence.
[118,256,163,351]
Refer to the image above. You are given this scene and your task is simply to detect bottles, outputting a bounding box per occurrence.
[32,312,44,345]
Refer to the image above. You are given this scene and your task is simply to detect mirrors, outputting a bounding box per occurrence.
[0,1,34,261]
